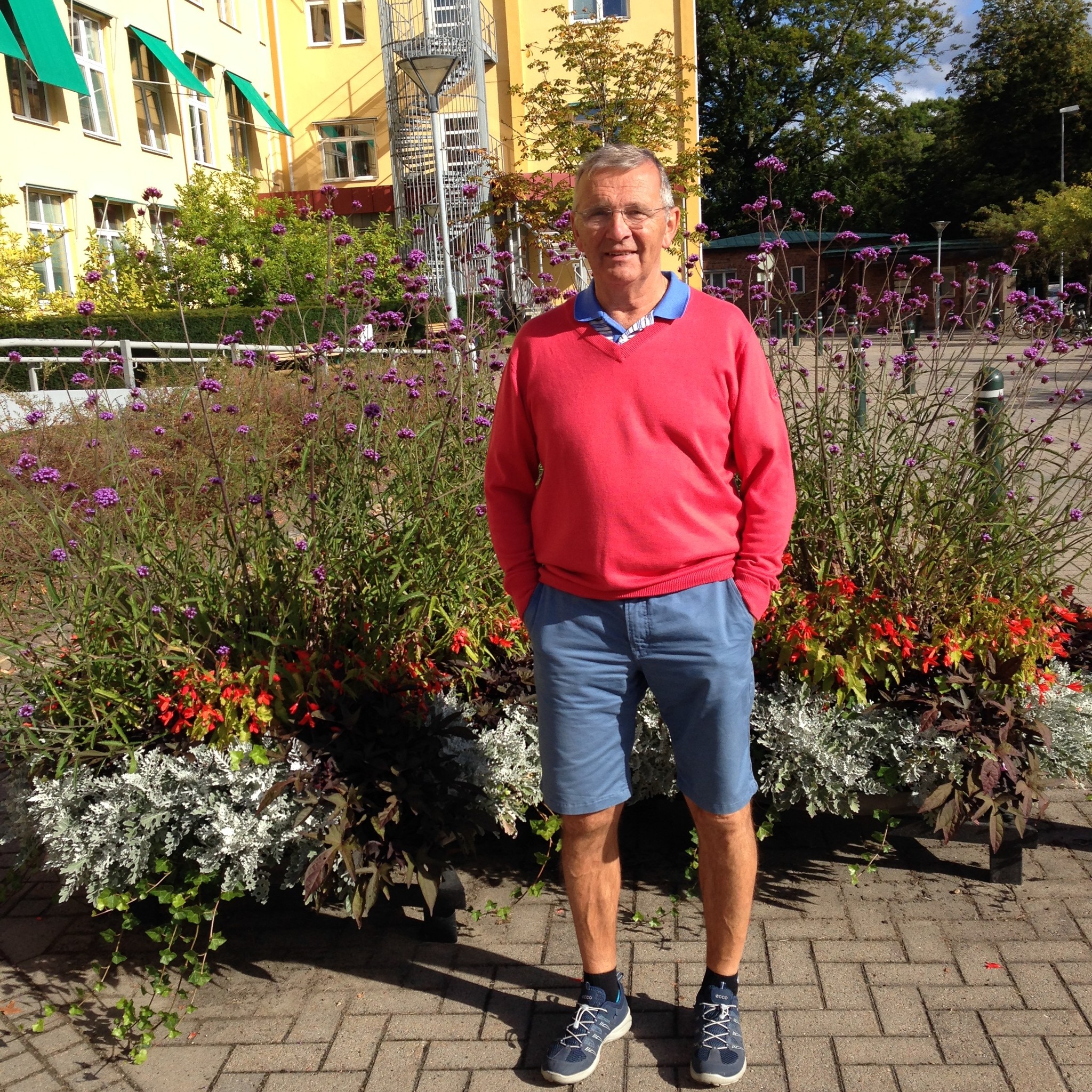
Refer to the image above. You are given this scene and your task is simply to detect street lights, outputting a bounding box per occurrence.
[394,54,463,324]
[929,220,952,337]
[1059,102,1081,316]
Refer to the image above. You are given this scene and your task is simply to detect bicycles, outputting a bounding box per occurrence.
[984,300,1092,344]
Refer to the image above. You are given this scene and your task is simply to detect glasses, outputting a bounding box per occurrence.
[572,206,669,229]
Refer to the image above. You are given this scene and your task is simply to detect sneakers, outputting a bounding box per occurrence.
[691,982,747,1085]
[540,971,633,1084]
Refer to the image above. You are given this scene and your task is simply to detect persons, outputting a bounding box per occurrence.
[483,143,796,1086]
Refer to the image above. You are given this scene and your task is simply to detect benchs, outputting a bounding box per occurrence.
[270,344,341,370]
[425,322,448,336]
[374,330,407,348]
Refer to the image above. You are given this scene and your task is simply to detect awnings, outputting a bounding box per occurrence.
[357,130,373,149]
[0,0,91,97]
[128,24,214,99]
[323,125,360,162]
[226,70,295,138]
